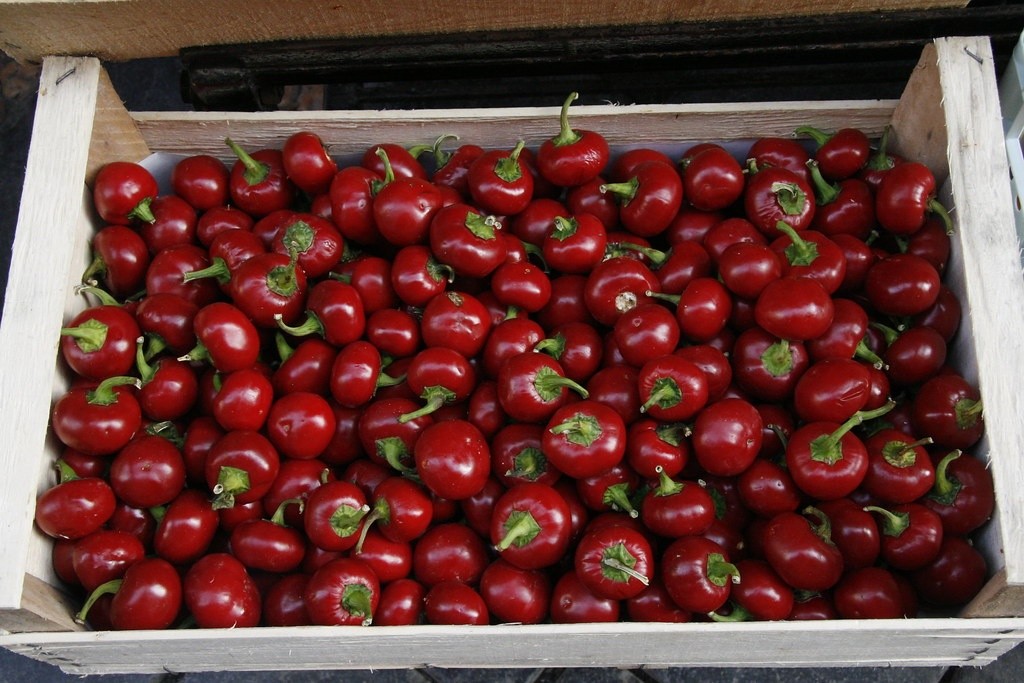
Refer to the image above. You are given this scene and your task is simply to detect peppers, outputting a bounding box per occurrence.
[37,91,996,631]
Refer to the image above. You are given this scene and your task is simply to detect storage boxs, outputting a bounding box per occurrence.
[0,31,1023,681]
